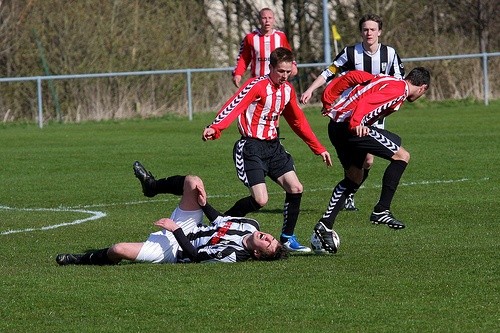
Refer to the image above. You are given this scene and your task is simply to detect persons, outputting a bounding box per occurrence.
[231,6,298,139]
[55,161,292,267]
[313,66,432,255]
[299,11,406,212]
[200,46,333,255]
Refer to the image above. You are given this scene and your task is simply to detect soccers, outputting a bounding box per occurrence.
[311,229,340,254]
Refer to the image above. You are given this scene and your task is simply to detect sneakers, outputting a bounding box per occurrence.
[343,192,356,210]
[280,233,312,253]
[56,254,87,265]
[132,160,156,198]
[313,223,338,253]
[370,209,405,229]
